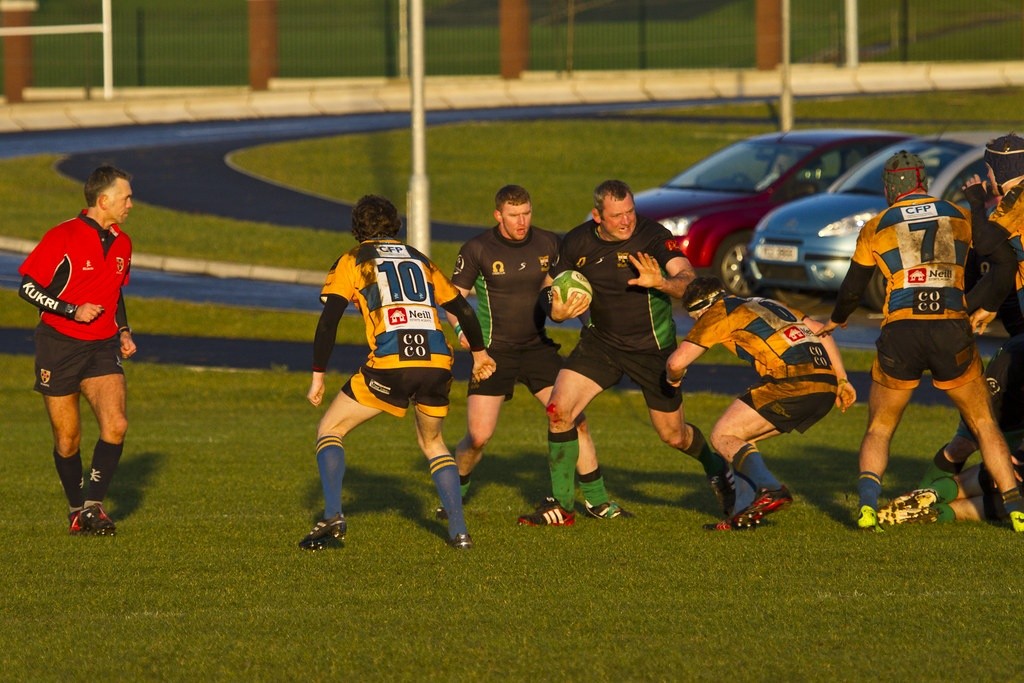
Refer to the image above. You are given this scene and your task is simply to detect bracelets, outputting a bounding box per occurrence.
[454,321,463,339]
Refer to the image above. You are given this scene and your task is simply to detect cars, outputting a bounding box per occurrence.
[746,133,1024,312]
[586,129,917,299]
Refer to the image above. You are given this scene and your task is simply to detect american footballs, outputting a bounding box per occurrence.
[550,271,593,308]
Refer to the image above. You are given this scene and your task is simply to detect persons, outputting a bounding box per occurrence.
[666,276,856,532]
[814,150,1024,533]
[919,130,1024,490]
[754,153,798,190]
[435,185,639,519]
[18,165,138,534]
[298,194,497,549]
[518,180,739,527]
[877,462,1024,528]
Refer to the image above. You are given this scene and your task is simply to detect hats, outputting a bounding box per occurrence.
[882,150,929,204]
[983,134,1024,187]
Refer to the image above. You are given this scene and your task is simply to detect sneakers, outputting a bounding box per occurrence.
[733,484,793,528]
[716,519,735,530]
[437,504,448,519]
[877,488,938,525]
[1005,502,1024,534]
[82,502,117,535]
[298,512,346,548]
[857,504,885,534]
[910,507,936,525]
[517,496,575,527]
[68,509,87,536]
[453,532,474,549]
[584,500,635,519]
[707,453,736,515]
